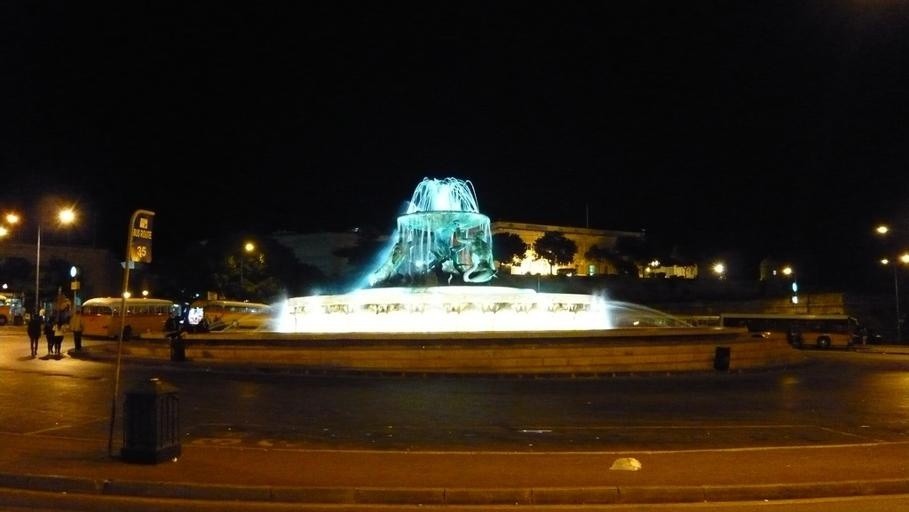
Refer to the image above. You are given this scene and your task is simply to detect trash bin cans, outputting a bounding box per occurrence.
[122,378,182,464]
[171,339,185,362]
[714,347,730,370]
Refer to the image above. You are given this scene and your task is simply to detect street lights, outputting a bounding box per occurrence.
[781,266,798,313]
[239,239,255,303]
[36,203,75,317]
[68,264,83,351]
[876,220,901,345]
[651,259,660,278]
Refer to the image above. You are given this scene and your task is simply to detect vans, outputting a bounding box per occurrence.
[6,297,21,311]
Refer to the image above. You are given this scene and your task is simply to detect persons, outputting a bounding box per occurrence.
[846,334,857,352]
[163,310,186,331]
[27,314,41,356]
[861,327,868,346]
[68,311,84,350]
[874,327,883,344]
[53,320,64,355]
[43,319,55,355]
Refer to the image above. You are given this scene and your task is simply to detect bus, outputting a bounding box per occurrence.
[719,310,868,351]
[0,294,10,324]
[557,267,577,276]
[76,295,178,340]
[187,298,279,330]
